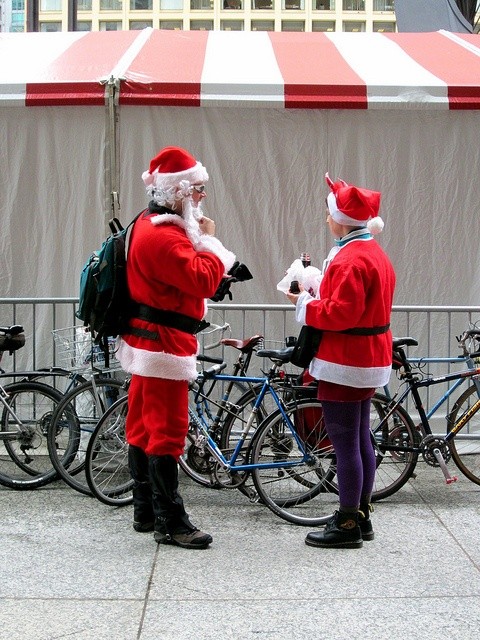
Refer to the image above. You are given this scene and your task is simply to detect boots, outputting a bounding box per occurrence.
[305,510,363,548]
[359,504,374,541]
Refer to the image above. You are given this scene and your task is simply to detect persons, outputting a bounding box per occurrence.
[125,146,237,546]
[285,171,396,550]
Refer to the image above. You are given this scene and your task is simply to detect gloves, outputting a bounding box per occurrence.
[210,261,253,301]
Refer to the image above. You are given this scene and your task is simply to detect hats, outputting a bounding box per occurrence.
[141,146,209,191]
[325,186,386,234]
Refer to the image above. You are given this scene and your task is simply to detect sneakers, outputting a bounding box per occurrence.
[153,528,213,548]
[132,510,189,532]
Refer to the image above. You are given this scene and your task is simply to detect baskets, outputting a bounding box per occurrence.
[54,323,119,371]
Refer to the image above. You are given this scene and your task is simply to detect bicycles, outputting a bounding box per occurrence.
[85,346,361,527]
[2,341,264,480]
[276,320,479,492]
[308,336,480,504]
[0,325,82,491]
[46,316,338,506]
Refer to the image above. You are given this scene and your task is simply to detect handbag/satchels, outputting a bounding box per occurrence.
[290,326,321,367]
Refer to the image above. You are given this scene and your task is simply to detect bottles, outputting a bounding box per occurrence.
[299,251,316,304]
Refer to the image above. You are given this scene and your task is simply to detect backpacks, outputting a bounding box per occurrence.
[74,208,148,337]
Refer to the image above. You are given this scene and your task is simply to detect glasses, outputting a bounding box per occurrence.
[192,184,205,193]
